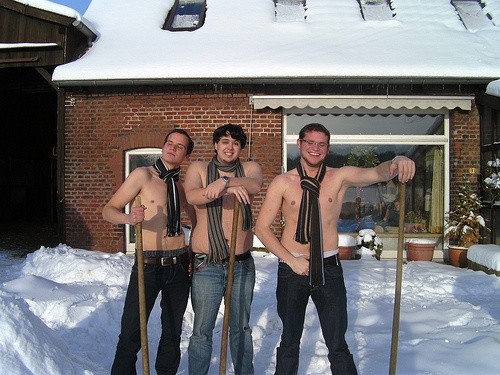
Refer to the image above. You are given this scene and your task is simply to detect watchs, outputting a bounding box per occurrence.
[220,176,229,187]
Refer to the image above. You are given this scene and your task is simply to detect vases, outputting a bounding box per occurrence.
[405,242,435,261]
[337,246,356,260]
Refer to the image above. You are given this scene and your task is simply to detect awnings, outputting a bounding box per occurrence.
[249,95,475,110]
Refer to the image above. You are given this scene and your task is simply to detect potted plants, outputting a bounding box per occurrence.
[442,179,491,268]
[405,211,427,232]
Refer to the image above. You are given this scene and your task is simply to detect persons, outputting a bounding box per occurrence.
[102,129,197,375]
[254,122,415,375]
[377,200,401,228]
[184,123,262,375]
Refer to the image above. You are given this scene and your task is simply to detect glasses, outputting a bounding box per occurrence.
[299,139,329,148]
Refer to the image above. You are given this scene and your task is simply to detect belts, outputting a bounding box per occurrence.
[193,250,251,261]
[136,255,189,266]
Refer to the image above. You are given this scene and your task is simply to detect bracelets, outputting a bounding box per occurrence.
[392,155,400,164]
